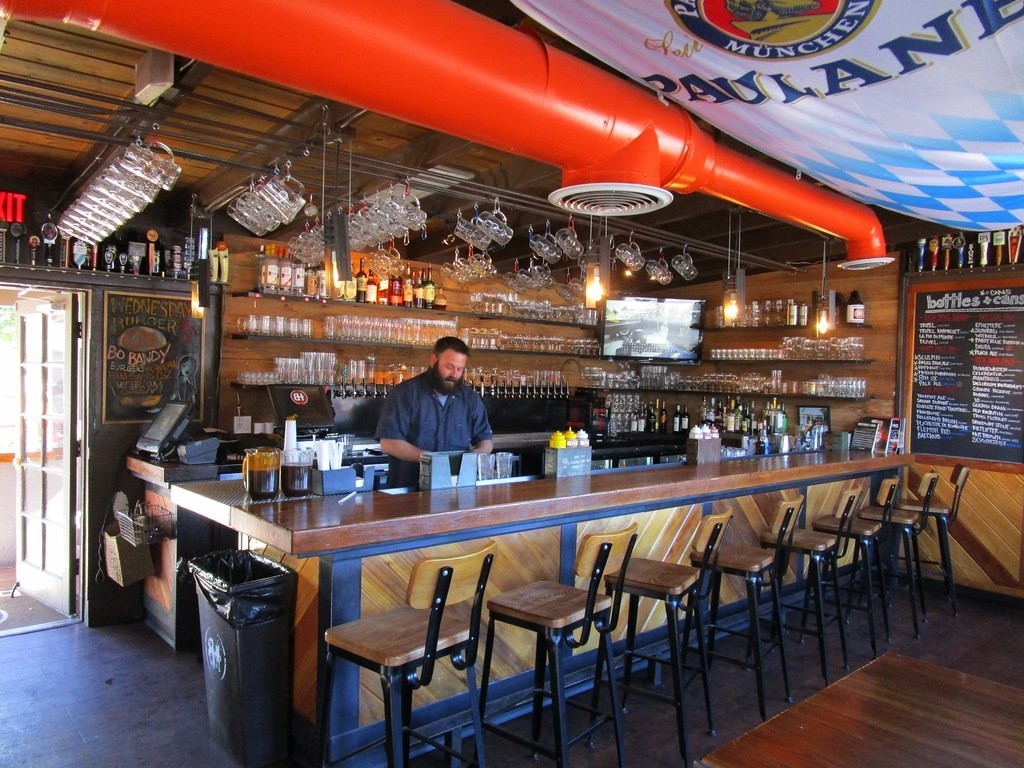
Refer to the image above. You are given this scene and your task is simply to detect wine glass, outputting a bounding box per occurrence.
[504,292,584,324]
[783,336,864,360]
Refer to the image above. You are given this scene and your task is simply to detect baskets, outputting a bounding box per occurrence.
[117,504,174,547]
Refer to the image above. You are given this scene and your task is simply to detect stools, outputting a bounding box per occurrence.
[746,491,864,689]
[812,476,904,659]
[324,551,493,768]
[692,493,805,722]
[478,523,642,767]
[859,467,972,618]
[595,504,734,768]
[844,468,940,641]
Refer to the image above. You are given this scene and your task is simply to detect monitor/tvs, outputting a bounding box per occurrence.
[267,384,335,429]
[600,295,705,366]
[137,401,193,453]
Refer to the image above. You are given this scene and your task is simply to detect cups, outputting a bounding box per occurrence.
[56,141,181,245]
[236,314,313,339]
[555,227,583,260]
[672,254,698,280]
[468,292,483,314]
[241,446,314,499]
[254,422,275,435]
[644,258,673,285]
[502,265,556,294]
[440,252,497,287]
[529,233,562,263]
[584,309,598,325]
[555,278,585,303]
[322,315,458,345]
[710,348,782,359]
[478,453,512,481]
[460,327,599,354]
[362,249,404,280]
[285,192,427,267]
[615,242,645,271]
[238,352,868,399]
[454,209,513,249]
[226,174,306,236]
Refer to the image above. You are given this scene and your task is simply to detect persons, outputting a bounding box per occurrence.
[375,336,493,485]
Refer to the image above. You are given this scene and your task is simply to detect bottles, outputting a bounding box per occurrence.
[550,426,589,449]
[630,398,666,432]
[674,404,690,431]
[688,395,824,458]
[253,244,326,297]
[483,288,504,316]
[345,258,448,310]
[341,434,355,456]
[716,289,864,328]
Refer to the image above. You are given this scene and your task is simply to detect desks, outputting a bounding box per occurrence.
[692,650,1024,768]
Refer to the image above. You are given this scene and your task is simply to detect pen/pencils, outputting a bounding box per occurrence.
[338,491,360,502]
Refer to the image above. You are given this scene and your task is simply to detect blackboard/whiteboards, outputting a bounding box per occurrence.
[903,278,1024,477]
[103,290,206,423]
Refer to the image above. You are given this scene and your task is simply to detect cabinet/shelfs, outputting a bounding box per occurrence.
[225,285,640,390]
[641,317,872,397]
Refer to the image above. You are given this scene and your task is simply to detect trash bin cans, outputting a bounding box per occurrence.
[188,548,296,768]
